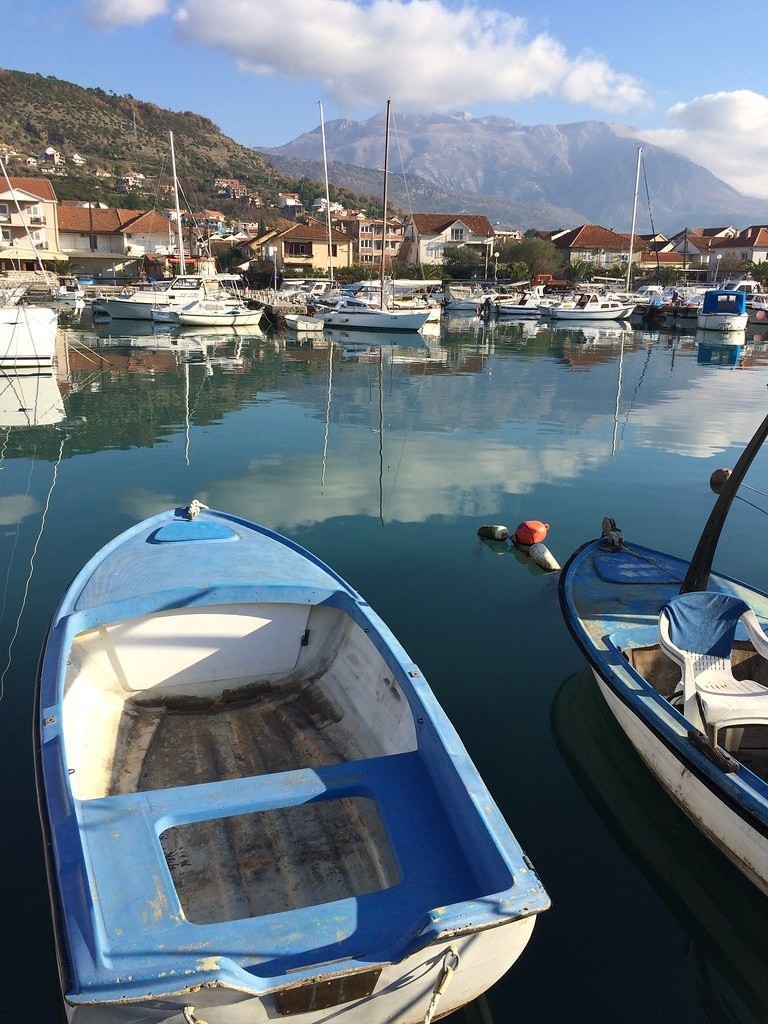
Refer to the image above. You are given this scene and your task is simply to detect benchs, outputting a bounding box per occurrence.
[78,748,482,975]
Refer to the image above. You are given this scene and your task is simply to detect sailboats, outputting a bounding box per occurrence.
[0,366,72,702]
[274,97,768,335]
[89,128,266,326]
[0,158,62,368]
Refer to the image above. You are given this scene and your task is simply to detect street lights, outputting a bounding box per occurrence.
[713,254,723,287]
[484,221,500,279]
[273,245,278,293]
[196,241,200,275]
[494,251,500,285]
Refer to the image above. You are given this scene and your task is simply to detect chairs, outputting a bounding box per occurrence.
[657,591,768,753]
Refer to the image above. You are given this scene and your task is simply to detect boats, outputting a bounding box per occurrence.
[57,298,85,321]
[695,288,750,334]
[56,274,85,301]
[283,312,326,331]
[554,412,768,895]
[30,497,554,1024]
[547,664,768,1024]
[694,328,746,371]
